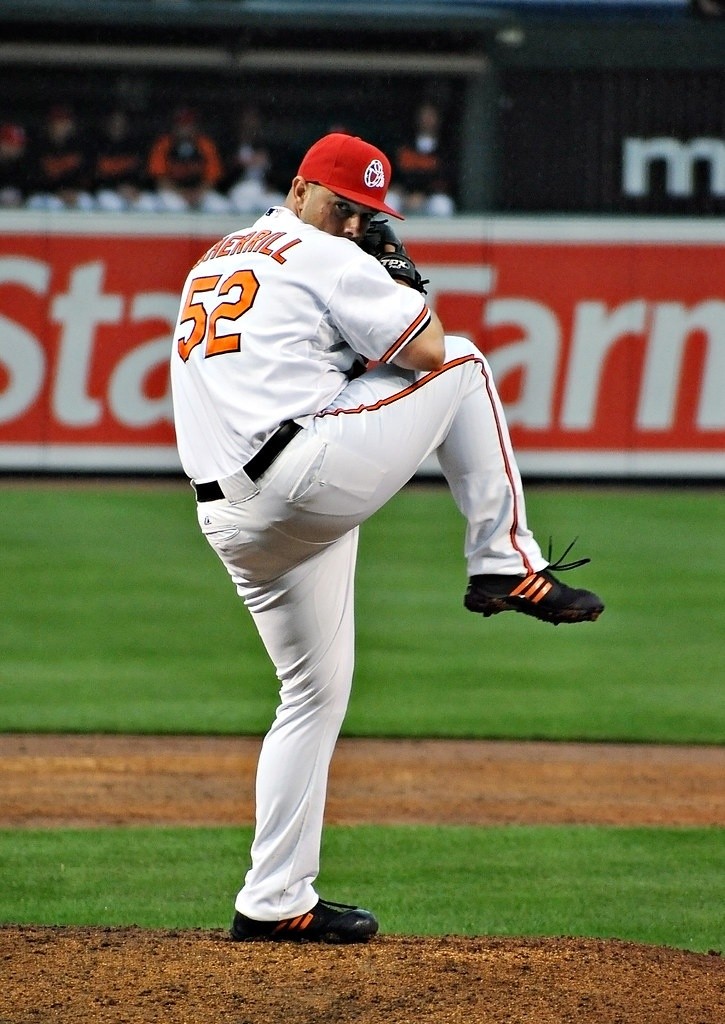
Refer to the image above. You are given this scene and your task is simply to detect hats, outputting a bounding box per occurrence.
[297,132,407,222]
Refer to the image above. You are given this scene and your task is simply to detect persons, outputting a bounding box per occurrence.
[169,132,606,944]
[1,100,458,216]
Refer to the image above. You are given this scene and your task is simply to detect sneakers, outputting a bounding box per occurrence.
[230,897,379,944]
[463,535,604,623]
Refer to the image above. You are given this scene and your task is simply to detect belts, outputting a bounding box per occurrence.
[194,419,304,503]
